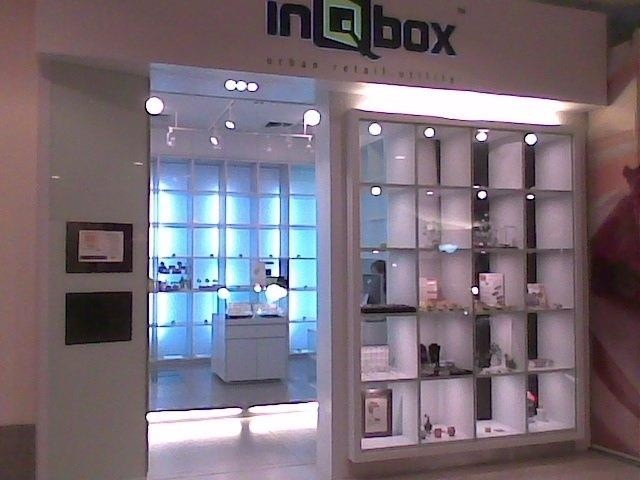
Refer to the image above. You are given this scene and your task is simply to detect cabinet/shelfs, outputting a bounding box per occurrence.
[348,107,584,464]
[150,152,317,365]
[212,313,288,384]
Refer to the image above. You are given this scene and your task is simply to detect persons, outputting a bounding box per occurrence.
[369,260,385,297]
